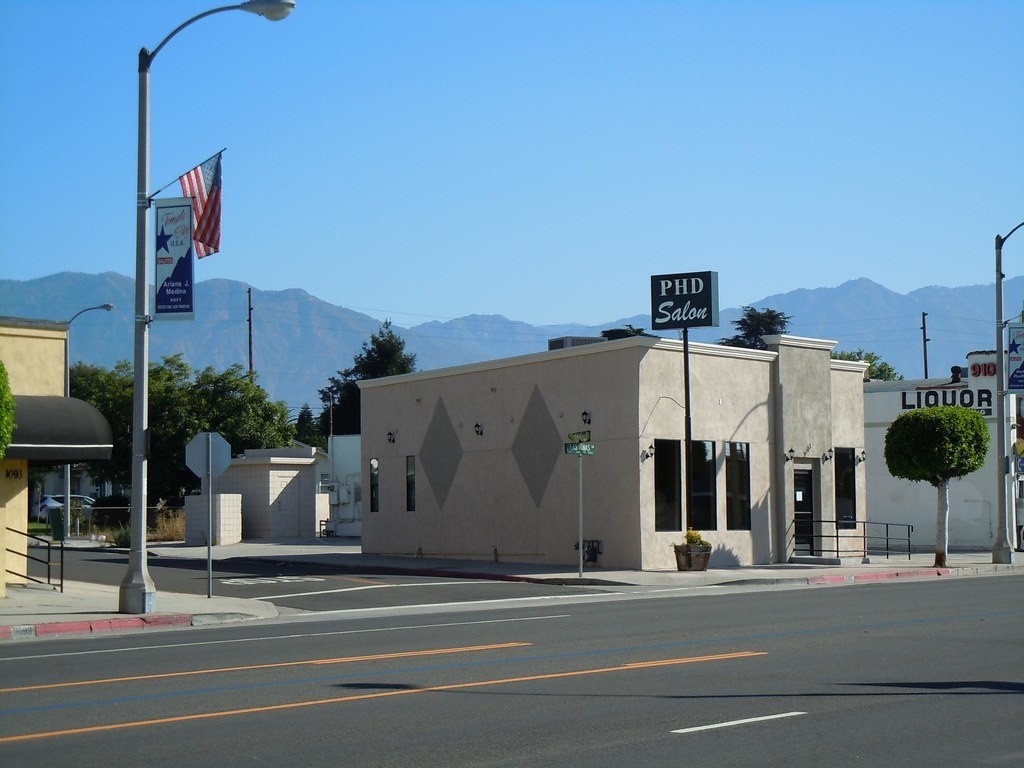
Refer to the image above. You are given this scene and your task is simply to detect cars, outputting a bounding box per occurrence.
[32,493,97,523]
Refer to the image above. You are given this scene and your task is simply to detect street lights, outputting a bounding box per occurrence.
[120,0,297,611]
[66,304,114,535]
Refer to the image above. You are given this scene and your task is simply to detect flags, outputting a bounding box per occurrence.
[179,151,223,260]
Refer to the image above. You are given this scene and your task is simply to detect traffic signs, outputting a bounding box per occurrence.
[565,443,595,455]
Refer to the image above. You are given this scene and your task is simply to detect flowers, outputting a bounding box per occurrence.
[684,527,703,545]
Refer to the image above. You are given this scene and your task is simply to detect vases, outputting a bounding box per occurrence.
[671,545,712,571]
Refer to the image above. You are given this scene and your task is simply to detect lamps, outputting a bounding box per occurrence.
[784,448,795,461]
[388,432,395,443]
[825,448,833,462]
[582,410,591,424]
[859,450,866,462]
[474,422,483,436]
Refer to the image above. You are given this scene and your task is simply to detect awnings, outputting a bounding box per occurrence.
[4,396,114,461]
